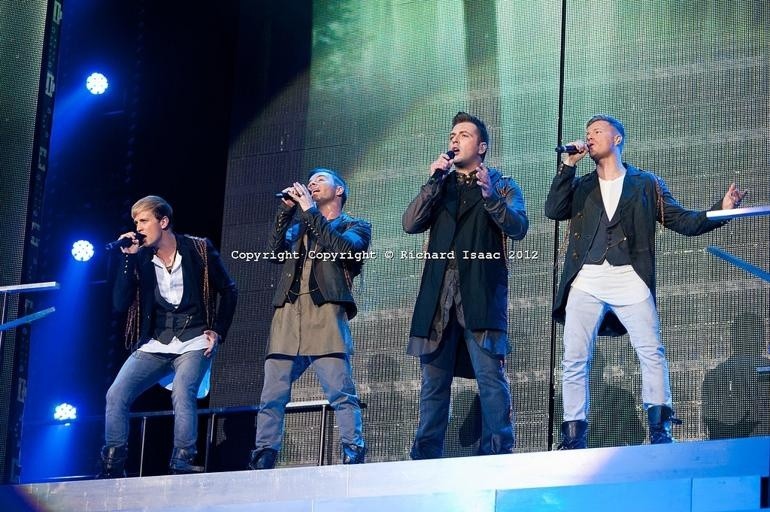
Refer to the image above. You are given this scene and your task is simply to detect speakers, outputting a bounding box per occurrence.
[169,445,198,475]
[646,404,679,444]
[557,419,589,450]
[95,446,128,479]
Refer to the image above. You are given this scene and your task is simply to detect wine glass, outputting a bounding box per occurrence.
[299,192,304,197]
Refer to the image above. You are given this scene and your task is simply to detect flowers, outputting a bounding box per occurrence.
[105,233,143,254]
[555,143,590,153]
[275,189,312,199]
[429,150,455,184]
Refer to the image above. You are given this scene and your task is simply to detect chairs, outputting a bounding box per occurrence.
[249,448,276,470]
[340,443,367,464]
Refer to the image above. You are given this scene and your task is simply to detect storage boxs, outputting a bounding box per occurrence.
[126,397,367,476]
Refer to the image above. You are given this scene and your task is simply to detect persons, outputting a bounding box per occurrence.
[401,112,533,459]
[92,197,238,479]
[248,168,372,470]
[543,117,747,449]
